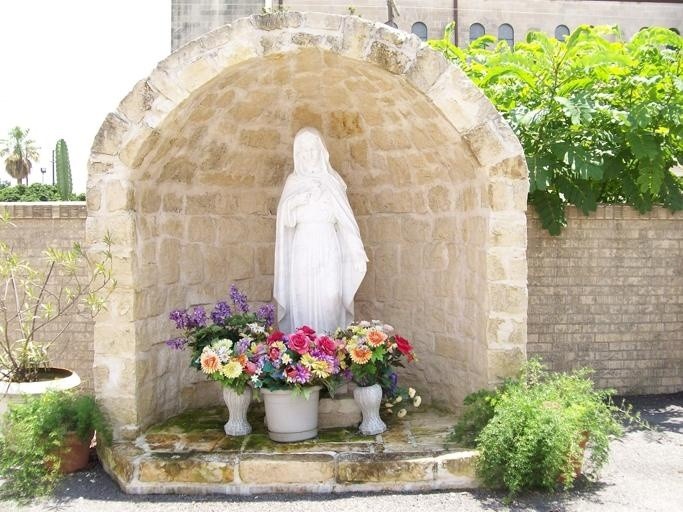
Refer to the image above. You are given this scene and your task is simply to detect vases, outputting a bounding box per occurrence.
[352,384,387,435]
[224,387,252,436]
[260,387,323,444]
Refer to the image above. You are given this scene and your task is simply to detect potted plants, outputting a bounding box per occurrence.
[0,388,112,505]
[441,355,651,506]
[0,209,118,435]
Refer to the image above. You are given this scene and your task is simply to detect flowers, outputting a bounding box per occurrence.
[168,289,421,417]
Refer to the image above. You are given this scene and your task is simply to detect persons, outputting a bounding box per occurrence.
[270,122,369,348]
